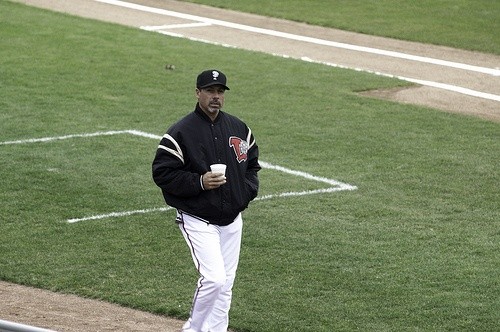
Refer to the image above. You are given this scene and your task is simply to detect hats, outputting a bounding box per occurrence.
[197,70,230,91]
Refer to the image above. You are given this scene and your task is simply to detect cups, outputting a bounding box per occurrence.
[210,164,226,178]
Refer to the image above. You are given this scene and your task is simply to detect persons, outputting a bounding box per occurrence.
[152,70,262,332]
